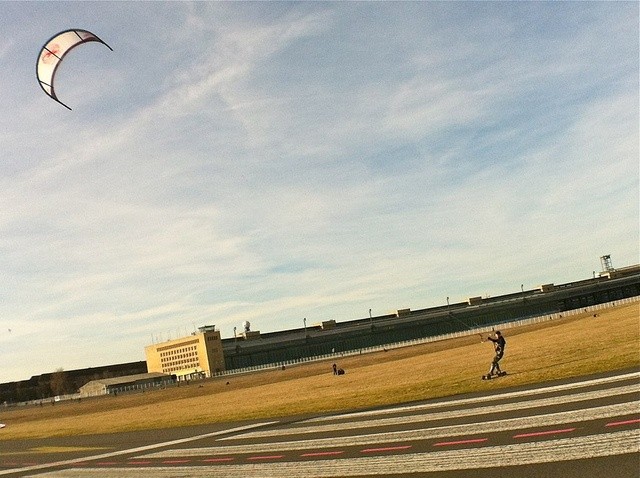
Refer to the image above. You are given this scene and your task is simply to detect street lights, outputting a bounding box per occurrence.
[522,284,524,297]
[304,318,308,339]
[233,327,237,343]
[593,271,596,283]
[369,308,373,323]
[447,297,451,311]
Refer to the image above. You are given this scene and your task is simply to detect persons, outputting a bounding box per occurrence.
[333,363,337,375]
[486,331,506,376]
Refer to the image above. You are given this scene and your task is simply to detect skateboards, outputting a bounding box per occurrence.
[482,371,506,380]
